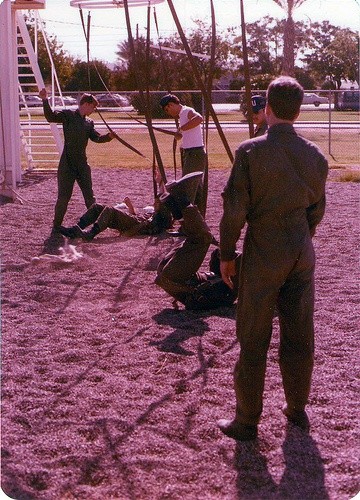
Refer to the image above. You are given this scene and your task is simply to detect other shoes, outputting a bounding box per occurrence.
[166,227,192,238]
[281,401,306,424]
[159,191,191,212]
[165,171,203,194]
[52,225,65,233]
[217,418,256,440]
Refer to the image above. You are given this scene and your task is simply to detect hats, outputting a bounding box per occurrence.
[244,95,266,115]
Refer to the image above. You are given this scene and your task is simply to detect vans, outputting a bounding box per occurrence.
[338,91,360,112]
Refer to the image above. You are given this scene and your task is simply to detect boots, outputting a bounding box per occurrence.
[76,224,100,243]
[60,218,87,239]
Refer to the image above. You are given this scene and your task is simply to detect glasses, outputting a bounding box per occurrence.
[253,106,265,114]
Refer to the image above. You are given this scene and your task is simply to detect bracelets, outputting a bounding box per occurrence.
[178,129,183,133]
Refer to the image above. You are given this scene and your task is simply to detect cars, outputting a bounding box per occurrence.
[302,93,329,107]
[19,94,131,107]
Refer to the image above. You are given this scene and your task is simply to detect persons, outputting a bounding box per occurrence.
[151,170,243,314]
[38,88,118,238]
[211,75,329,441]
[56,193,172,242]
[244,95,269,138]
[158,93,207,222]
